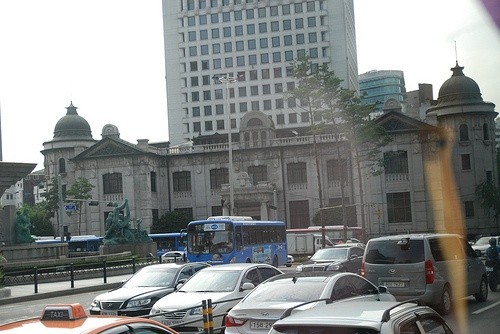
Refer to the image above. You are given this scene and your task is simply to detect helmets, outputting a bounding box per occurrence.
[489,238,496,245]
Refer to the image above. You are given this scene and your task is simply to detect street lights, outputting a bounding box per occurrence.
[211,73,245,216]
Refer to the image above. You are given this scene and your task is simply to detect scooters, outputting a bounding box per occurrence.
[485,260,500,292]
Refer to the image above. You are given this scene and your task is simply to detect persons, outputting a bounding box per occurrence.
[106,199,127,238]
[14,208,33,242]
[486,238,500,263]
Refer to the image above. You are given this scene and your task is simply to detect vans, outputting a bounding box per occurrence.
[360,234,489,316]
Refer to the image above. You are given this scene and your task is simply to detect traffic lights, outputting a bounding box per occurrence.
[66,232,71,240]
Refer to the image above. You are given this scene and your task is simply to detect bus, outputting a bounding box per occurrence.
[179,217,289,269]
[111,232,188,258]
[32,234,105,257]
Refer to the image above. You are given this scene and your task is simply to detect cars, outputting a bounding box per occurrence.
[267,298,455,334]
[224,271,398,334]
[295,238,365,275]
[0,302,180,334]
[148,262,286,334]
[88,262,213,319]
[470,236,500,257]
[161,251,185,262]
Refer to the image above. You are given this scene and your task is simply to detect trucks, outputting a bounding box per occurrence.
[286,233,335,256]
